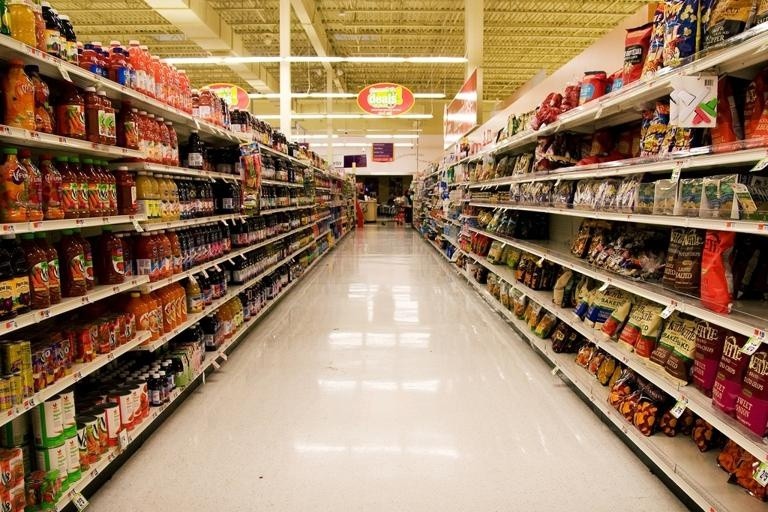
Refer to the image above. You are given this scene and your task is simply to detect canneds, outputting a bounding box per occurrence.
[0,381,149,502]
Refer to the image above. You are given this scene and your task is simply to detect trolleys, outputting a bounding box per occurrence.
[379,203,405,226]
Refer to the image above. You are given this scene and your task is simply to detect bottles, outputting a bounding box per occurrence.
[0,0,354,512]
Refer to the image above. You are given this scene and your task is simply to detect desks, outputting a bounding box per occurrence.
[360,198,377,223]
